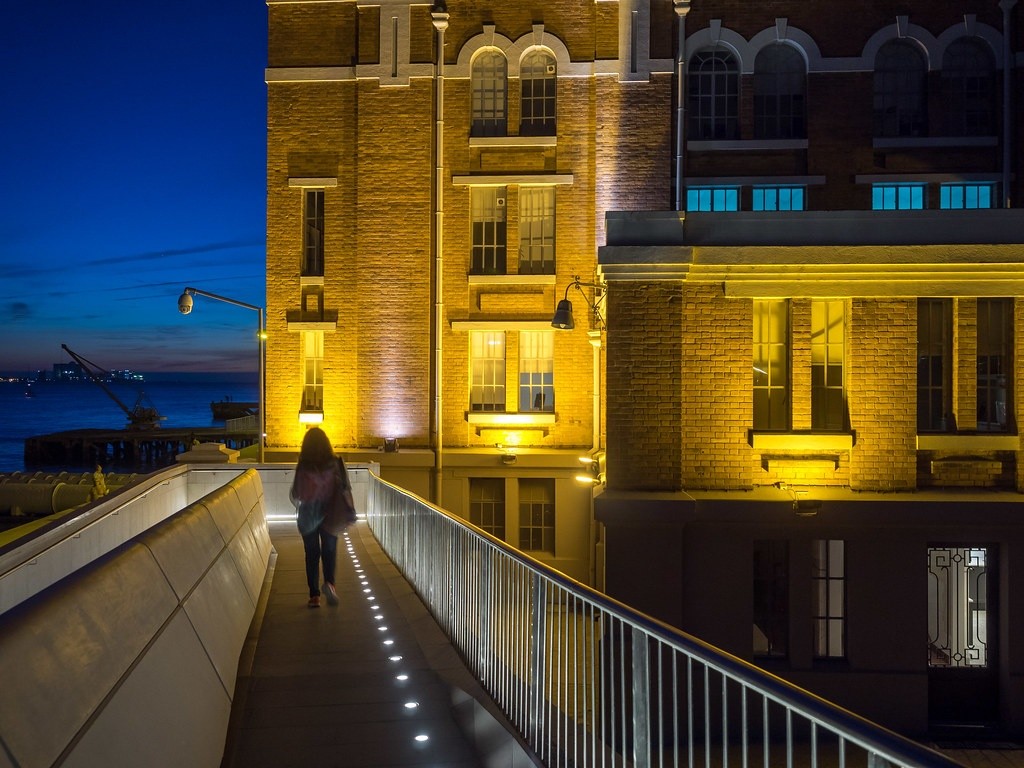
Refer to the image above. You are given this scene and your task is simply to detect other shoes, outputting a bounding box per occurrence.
[308,597,320,606]
[325,583,339,604]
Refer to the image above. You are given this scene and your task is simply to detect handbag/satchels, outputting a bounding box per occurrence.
[324,457,354,537]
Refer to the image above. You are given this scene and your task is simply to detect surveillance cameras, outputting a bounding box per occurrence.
[178,294,193,314]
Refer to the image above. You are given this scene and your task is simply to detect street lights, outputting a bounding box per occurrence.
[178,288,265,463]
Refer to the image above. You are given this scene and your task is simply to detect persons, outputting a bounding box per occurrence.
[292,428,356,608]
[87,464,106,503]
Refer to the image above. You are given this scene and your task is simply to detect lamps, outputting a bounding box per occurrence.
[384,437,399,453]
[793,500,822,517]
[551,280,607,333]
[503,454,518,464]
